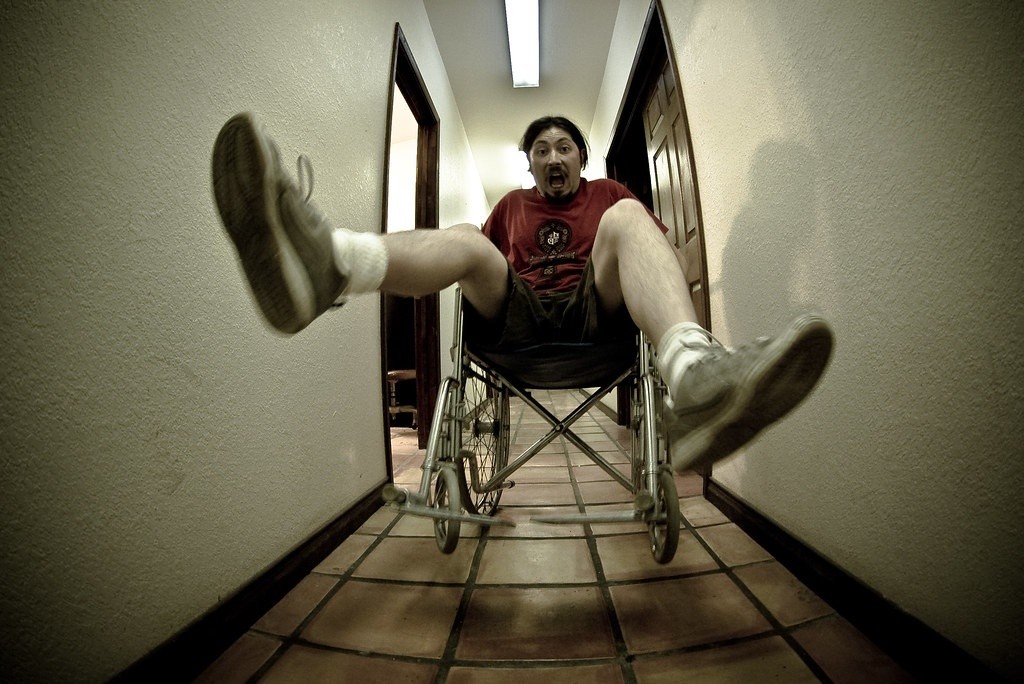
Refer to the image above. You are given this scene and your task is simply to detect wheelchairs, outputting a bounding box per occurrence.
[378,285,682,565]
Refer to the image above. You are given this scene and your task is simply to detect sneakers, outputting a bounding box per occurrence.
[667,313,837,476]
[210,112,345,335]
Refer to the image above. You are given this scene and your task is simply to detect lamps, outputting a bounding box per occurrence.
[504,0,540,88]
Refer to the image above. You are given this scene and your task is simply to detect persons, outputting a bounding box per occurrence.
[210,111,835,476]
